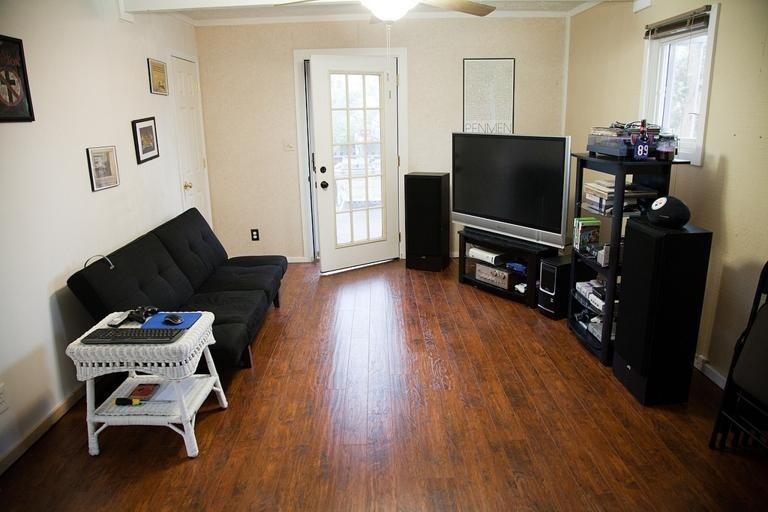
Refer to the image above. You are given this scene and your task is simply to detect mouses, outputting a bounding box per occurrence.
[162,314,184,325]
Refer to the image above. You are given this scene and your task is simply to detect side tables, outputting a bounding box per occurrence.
[65,308,229,457]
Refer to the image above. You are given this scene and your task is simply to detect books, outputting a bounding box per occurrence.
[589,322,616,342]
[576,279,616,310]
[582,179,656,214]
[573,216,600,254]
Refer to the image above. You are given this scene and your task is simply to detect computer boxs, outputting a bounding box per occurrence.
[537,254,597,321]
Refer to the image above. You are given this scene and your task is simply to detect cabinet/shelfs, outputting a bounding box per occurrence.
[565,153,674,367]
[458,225,558,310]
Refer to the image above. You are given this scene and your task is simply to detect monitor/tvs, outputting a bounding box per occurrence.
[449,132,573,249]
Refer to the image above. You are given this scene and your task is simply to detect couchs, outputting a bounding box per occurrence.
[66,207,289,394]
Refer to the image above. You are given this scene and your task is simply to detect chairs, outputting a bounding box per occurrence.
[707,259,768,461]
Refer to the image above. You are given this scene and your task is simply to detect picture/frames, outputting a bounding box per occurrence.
[85,145,120,194]
[0,33,35,124]
[131,116,160,166]
[463,60,514,135]
[146,57,170,97]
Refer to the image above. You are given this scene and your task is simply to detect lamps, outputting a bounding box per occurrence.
[358,0,417,24]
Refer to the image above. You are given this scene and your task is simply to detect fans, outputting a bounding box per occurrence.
[421,1,496,21]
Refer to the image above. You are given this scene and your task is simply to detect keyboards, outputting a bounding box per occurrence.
[80,327,184,344]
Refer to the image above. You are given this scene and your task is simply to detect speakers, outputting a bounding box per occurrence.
[404,172,451,273]
[643,195,692,230]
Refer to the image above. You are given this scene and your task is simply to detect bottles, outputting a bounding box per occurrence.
[634,120,649,161]
[655,132,677,160]
